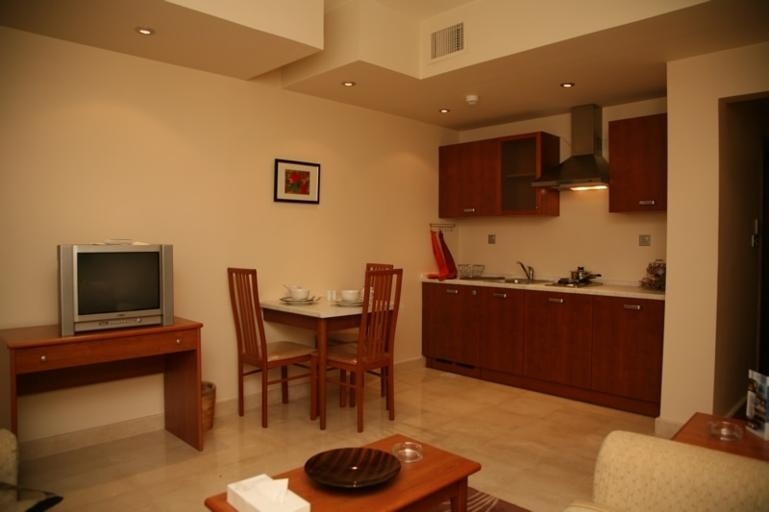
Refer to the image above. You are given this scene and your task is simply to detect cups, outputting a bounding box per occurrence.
[393,413,425,463]
[458,263,485,278]
[289,287,309,300]
[341,289,361,303]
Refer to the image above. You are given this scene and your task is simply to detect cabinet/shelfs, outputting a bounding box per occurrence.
[417,280,664,405]
[499,132,561,217]
[437,144,499,216]
[609,113,667,213]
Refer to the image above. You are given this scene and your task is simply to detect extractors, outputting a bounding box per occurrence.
[532,104,609,192]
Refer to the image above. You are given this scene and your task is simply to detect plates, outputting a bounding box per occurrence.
[301,443,400,491]
[279,296,320,304]
[331,300,365,307]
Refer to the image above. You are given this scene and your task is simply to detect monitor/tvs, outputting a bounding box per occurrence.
[57,244,174,336]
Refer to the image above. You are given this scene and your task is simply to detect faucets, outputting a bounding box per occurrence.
[517,260,533,279]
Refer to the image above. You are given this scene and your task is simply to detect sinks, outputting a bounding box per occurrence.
[483,278,554,285]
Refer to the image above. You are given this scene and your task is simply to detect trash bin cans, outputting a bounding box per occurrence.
[200,380,216,431]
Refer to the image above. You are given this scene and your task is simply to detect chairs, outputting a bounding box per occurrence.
[557,427,769,511]
[226,260,404,433]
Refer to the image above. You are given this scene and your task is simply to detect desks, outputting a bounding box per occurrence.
[671,411,768,460]
[0,313,204,457]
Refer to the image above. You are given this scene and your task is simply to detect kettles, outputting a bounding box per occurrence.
[569,266,602,282]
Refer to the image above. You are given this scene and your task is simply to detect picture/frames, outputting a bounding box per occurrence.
[274,158,321,204]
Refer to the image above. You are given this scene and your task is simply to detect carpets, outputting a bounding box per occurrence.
[437,485,534,512]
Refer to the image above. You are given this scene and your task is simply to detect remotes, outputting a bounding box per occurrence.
[104,239,133,245]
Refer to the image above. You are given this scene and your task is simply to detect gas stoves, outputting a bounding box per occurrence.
[545,277,602,288]
